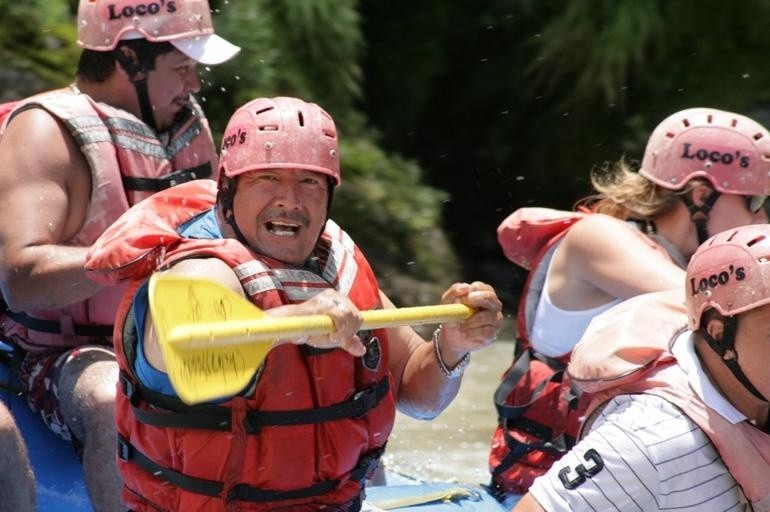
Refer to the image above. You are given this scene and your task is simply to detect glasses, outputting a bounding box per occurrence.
[745,196,769,214]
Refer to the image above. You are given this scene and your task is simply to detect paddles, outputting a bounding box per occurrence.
[148,271,477,405]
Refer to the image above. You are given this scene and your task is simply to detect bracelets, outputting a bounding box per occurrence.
[429,327,473,380]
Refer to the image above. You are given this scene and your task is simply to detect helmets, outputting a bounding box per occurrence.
[684,223,770,331]
[75,0,244,67]
[216,95,342,189]
[638,106,770,197]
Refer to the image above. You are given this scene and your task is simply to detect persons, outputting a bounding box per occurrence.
[483,106,770,496]
[86,97,505,511]
[1,1,254,510]
[510,222,770,512]
[0,402,40,511]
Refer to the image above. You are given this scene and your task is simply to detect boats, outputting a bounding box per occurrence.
[0,336,525,511]
